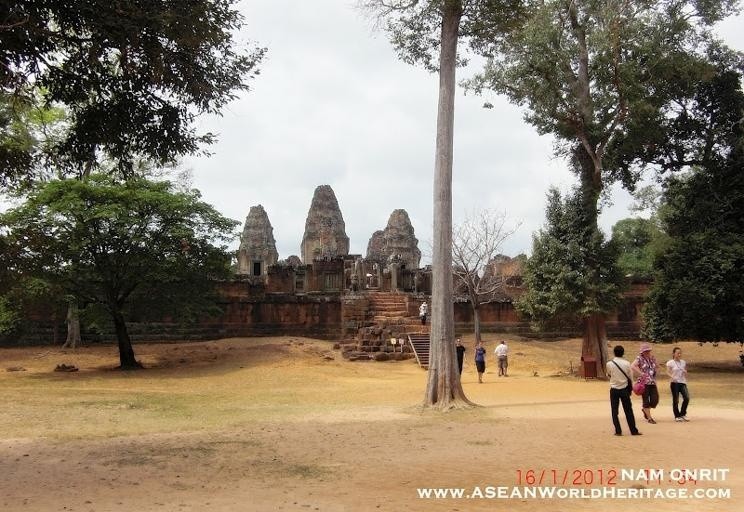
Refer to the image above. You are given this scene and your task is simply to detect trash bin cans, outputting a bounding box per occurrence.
[580,356,597,380]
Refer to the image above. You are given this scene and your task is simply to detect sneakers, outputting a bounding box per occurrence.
[642,408,655,424]
[675,415,690,421]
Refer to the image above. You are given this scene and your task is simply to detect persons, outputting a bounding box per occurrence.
[605,345,643,436]
[665,346,690,422]
[455,338,466,375]
[493,340,508,377]
[631,342,658,424]
[418,301,428,325]
[473,341,486,383]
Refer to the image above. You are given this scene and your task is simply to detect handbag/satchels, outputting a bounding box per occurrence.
[419,312,425,317]
[632,381,645,396]
[628,378,632,395]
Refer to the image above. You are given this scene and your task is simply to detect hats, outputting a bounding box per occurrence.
[422,301,427,306]
[639,344,652,354]
